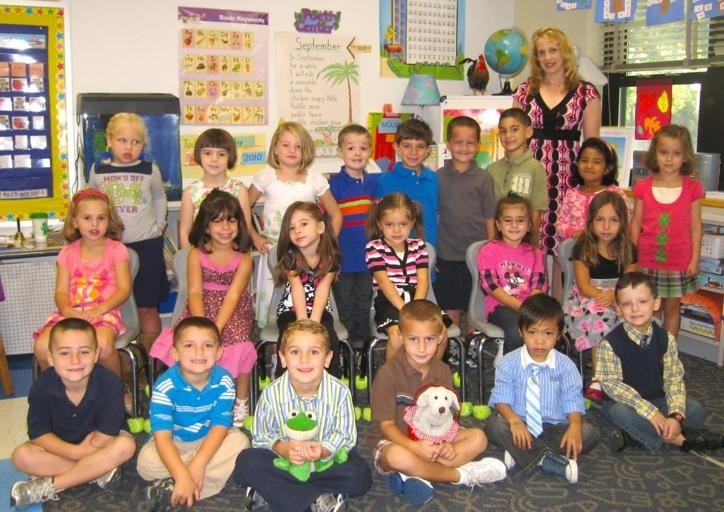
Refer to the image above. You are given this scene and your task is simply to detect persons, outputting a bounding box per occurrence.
[6,319,136,507]
[84,112,171,353]
[369,299,509,506]
[232,318,369,512]
[148,108,723,484]
[33,186,134,384]
[508,25,604,307]
[135,318,251,511]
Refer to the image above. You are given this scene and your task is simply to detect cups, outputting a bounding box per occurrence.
[31,213,49,243]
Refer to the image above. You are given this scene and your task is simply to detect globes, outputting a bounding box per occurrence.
[484,25,530,96]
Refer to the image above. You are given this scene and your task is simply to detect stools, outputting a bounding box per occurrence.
[0,276,15,395]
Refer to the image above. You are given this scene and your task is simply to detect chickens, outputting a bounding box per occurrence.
[459,54,489,96]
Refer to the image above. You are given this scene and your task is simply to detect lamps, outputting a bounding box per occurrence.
[402,75,441,144]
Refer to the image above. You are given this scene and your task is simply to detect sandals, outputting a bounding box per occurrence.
[124,384,142,416]
[233,397,250,430]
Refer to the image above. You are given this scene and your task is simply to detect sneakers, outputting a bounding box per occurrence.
[90,468,121,495]
[681,428,724,453]
[10,475,61,508]
[611,428,631,453]
[315,491,348,512]
[145,477,175,512]
[541,451,579,485]
[451,457,509,486]
[386,472,436,506]
[244,485,268,511]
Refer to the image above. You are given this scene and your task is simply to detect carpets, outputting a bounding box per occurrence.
[40,331,724,512]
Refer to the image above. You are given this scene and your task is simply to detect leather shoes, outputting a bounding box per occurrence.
[582,376,605,404]
